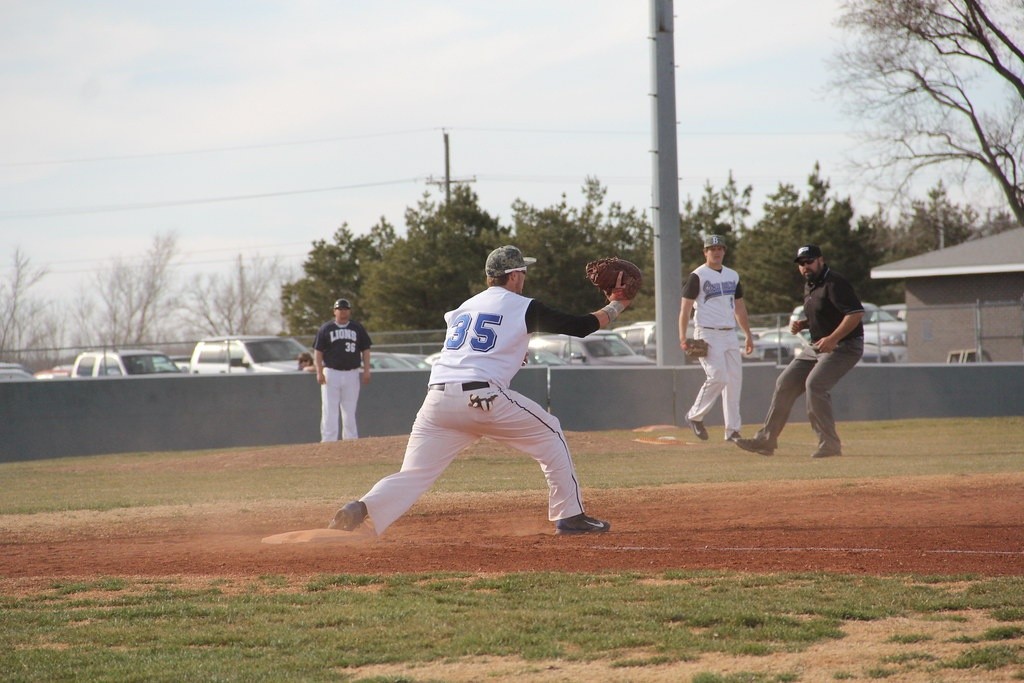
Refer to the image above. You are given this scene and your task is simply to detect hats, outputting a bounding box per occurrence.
[704,234,727,252]
[485,245,537,277]
[792,244,822,263]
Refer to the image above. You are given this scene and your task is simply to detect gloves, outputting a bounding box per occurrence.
[468,393,499,412]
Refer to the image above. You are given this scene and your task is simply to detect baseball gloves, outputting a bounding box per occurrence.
[586,258,640,301]
[686,338,707,360]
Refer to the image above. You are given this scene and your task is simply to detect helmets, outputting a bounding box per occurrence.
[334,299,351,308]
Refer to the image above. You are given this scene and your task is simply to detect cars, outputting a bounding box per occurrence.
[153,354,194,372]
[356,351,433,369]
[0,362,73,383]
[69,349,185,378]
[869,304,906,325]
[590,321,895,365]
[529,330,657,366]
[426,346,566,369]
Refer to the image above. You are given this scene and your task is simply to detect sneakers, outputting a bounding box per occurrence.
[728,431,742,444]
[811,447,842,458]
[328,500,369,531]
[736,438,773,456]
[685,412,709,440]
[554,513,611,535]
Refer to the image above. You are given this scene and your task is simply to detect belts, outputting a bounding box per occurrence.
[704,327,733,330]
[333,368,352,371]
[429,382,489,391]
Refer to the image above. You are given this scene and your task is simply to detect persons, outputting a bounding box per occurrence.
[312,299,372,444]
[678,234,754,442]
[328,244,641,535]
[734,244,865,458]
[298,352,314,370]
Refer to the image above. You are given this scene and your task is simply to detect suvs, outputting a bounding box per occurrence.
[189,337,314,376]
[788,301,904,362]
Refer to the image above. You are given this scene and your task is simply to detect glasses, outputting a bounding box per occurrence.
[799,256,820,266]
[517,269,526,275]
